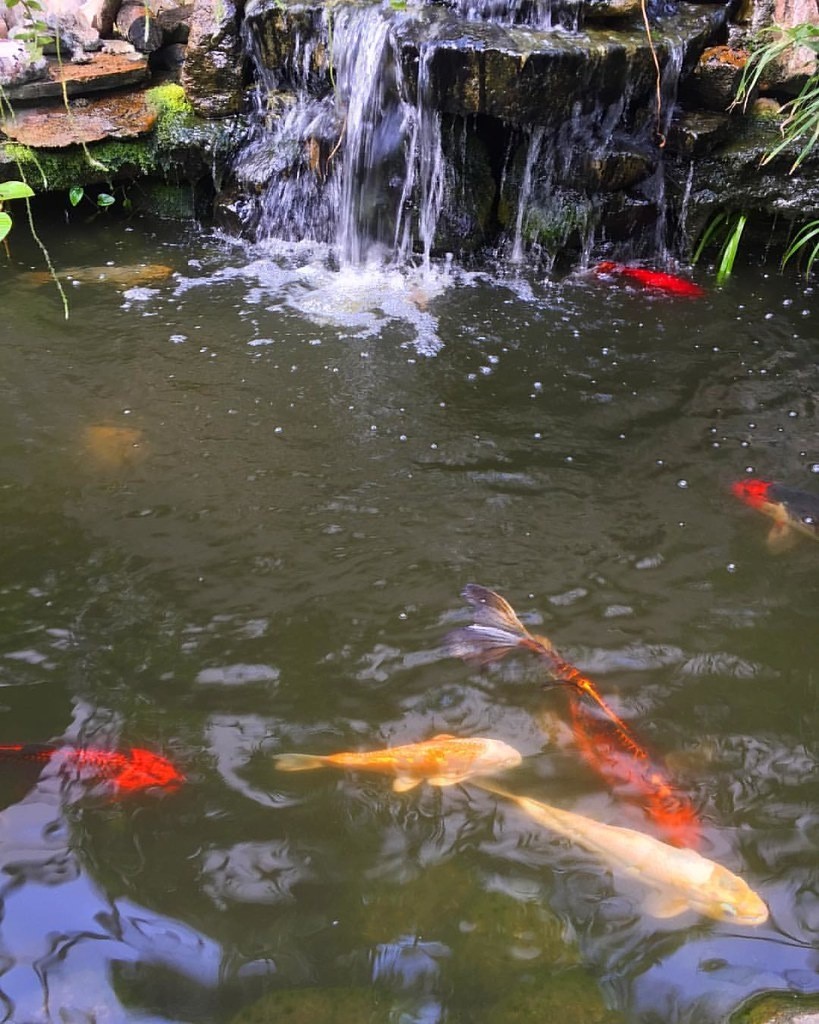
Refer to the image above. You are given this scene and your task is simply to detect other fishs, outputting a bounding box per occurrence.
[0,743,187,807]
[272,734,769,927]
[17,265,173,292]
[729,478,818,552]
[595,260,707,298]
[439,584,701,852]
